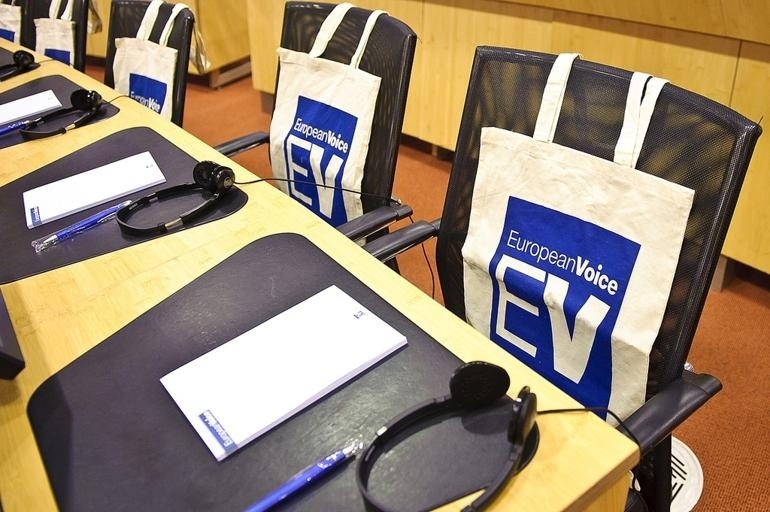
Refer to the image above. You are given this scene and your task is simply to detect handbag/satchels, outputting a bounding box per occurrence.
[0,1,22,46]
[34,17,75,67]
[269,46,382,249]
[112,37,179,122]
[461,124,696,429]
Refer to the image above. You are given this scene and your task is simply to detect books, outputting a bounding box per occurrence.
[157,282,408,464]
[22,149,167,231]
[0,88,63,128]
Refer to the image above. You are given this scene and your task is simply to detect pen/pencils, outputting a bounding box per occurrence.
[31,200,133,253]
[0,120,31,136]
[244,444,358,512]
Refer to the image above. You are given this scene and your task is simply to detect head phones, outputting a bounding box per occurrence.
[114,159,235,238]
[20,89,103,140]
[355,360,540,510]
[0,48,34,82]
